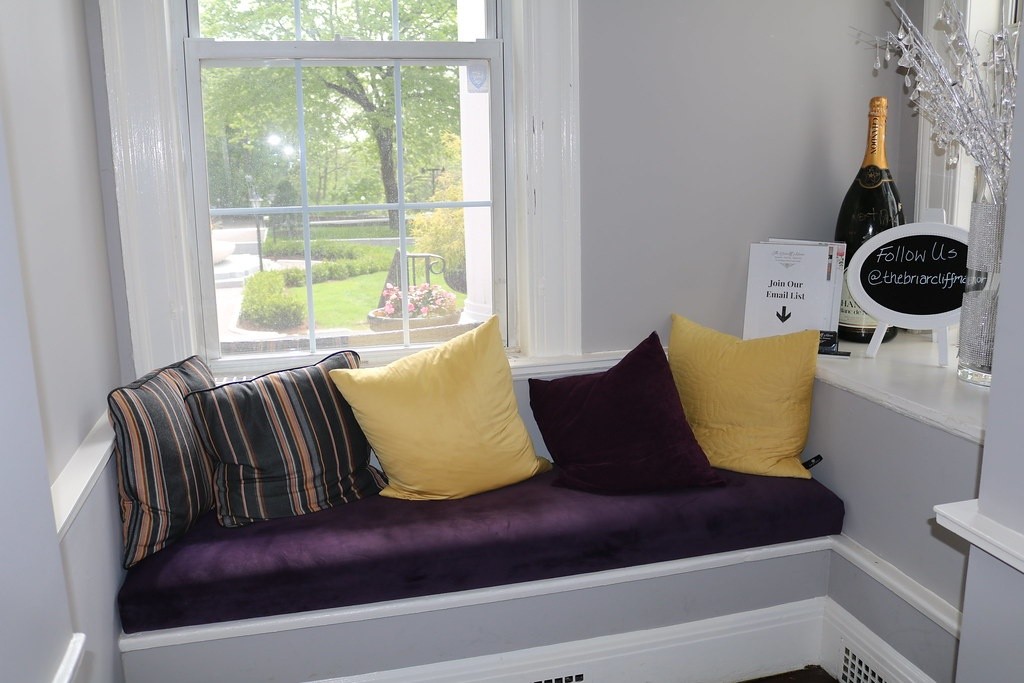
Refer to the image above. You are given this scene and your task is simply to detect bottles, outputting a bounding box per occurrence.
[833,96,908,344]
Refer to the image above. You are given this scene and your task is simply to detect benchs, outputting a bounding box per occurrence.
[114,468,845,683]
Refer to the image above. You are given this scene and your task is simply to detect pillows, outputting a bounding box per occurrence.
[668,313,820,479]
[183,349,386,527]
[107,355,217,569]
[528,331,721,496]
[328,316,553,499]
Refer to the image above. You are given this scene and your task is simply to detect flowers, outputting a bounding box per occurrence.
[382,283,456,317]
[850,1,1018,166]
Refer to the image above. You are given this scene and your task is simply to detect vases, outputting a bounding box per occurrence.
[957,166,1009,386]
[370,307,459,331]
